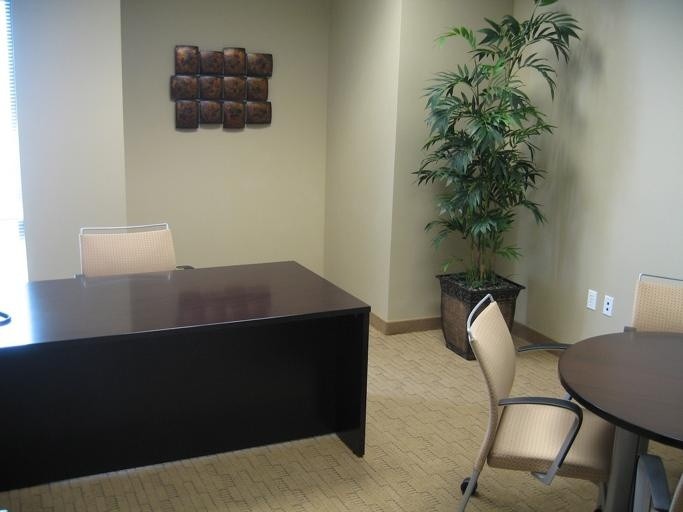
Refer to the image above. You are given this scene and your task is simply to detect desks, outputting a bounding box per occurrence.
[0,259,372,494]
[558,332,683,512]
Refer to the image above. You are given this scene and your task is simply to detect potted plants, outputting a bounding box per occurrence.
[410,0,586,360]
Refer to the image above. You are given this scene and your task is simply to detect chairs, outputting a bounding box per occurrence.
[624,273,683,332]
[74,223,194,278]
[456,294,616,512]
[632,454,683,512]
